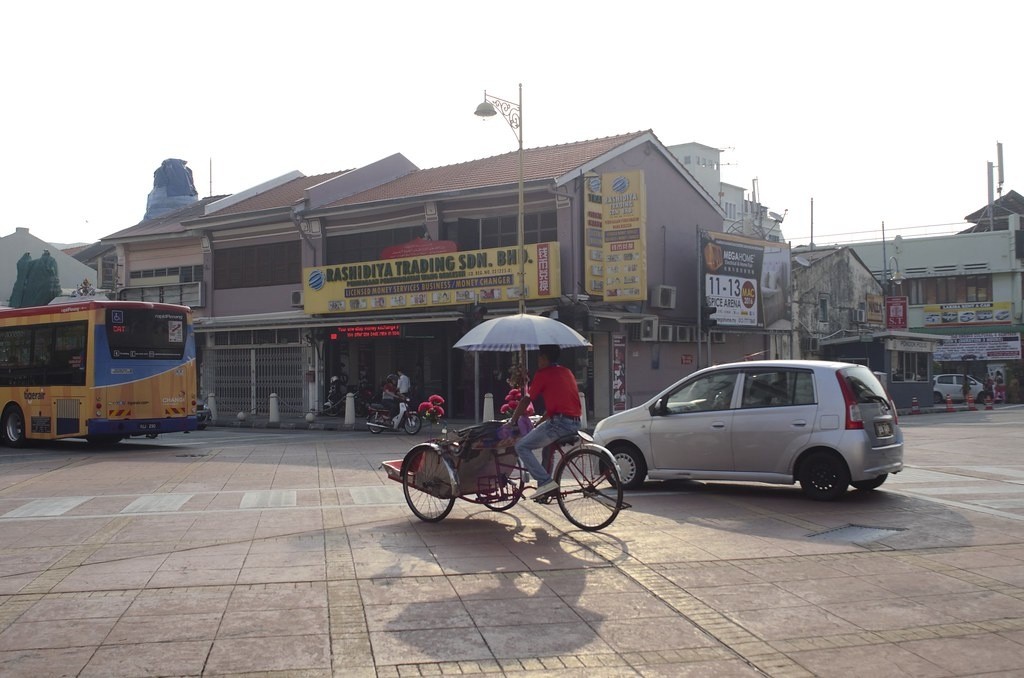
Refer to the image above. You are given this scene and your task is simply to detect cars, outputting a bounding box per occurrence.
[933,374,1003,405]
[196,398,212,431]
[590,359,904,502]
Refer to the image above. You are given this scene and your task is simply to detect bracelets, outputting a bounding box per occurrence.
[508,418,516,425]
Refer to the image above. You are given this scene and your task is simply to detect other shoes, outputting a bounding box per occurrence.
[529,481,559,499]
[383,422,391,426]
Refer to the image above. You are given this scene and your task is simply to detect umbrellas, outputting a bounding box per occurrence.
[452,312,593,393]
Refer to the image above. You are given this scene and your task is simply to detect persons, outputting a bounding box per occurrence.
[994,371,1005,403]
[960,378,971,399]
[982,373,994,401]
[9,356,18,363]
[396,368,410,395]
[502,344,584,499]
[382,374,405,426]
[1009,375,1019,403]
[415,363,422,383]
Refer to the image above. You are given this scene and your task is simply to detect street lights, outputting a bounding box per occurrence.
[473,83,526,313]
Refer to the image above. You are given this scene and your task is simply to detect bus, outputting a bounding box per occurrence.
[0,300,199,448]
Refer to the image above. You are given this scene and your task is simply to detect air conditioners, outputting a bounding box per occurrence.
[658,325,673,342]
[712,333,726,343]
[675,325,691,342]
[290,290,303,307]
[850,309,866,323]
[650,284,677,309]
[690,326,707,342]
[801,336,820,352]
[619,313,659,341]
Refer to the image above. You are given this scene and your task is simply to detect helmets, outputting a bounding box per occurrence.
[330,376,339,383]
[387,374,398,383]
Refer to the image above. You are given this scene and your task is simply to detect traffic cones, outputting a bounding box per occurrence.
[910,394,922,414]
[944,394,957,412]
[966,393,978,410]
[984,394,994,410]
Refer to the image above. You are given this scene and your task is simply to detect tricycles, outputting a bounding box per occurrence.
[382,412,633,532]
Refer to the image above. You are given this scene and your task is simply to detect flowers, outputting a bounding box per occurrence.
[417,395,445,442]
[499,388,536,420]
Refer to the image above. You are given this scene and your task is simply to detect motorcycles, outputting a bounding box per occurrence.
[366,395,422,435]
